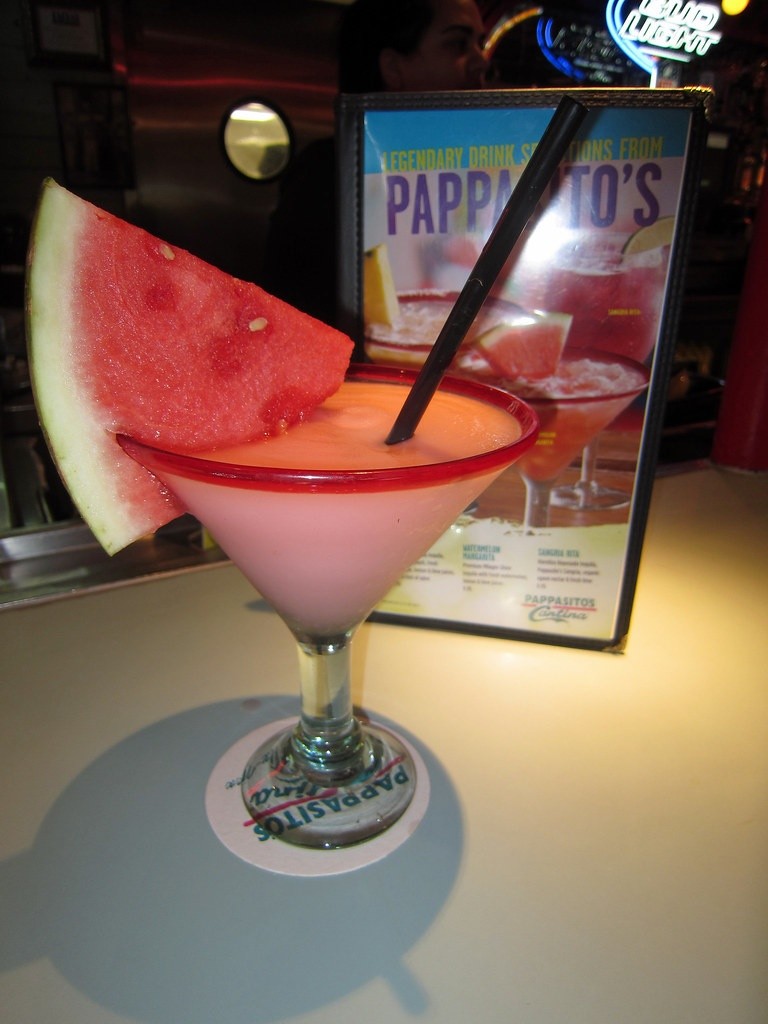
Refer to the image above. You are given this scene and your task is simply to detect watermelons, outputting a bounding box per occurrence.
[458,313,571,379]
[25,178,355,555]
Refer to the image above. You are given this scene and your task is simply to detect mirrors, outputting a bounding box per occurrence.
[222,99,295,182]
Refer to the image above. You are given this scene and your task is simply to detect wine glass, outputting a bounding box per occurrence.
[115,363,542,849]
[497,233,665,529]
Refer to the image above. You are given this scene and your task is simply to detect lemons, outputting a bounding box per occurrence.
[619,215,675,262]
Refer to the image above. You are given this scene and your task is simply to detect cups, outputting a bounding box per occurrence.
[368,285,538,369]
